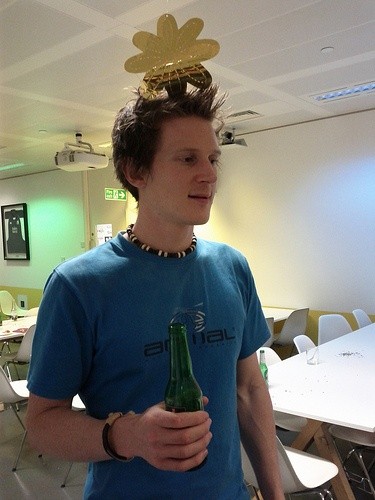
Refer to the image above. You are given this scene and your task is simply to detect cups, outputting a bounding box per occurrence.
[306,347,319,365]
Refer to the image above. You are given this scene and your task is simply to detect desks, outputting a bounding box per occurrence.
[261,307,295,323]
[0,315,37,357]
[267,322,375,500]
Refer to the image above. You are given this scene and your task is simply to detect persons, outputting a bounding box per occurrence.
[25,81,286,500]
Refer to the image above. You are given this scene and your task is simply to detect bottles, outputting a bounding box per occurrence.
[165,323,204,414]
[258,351,269,389]
[11,300,17,320]
[0,305,2,326]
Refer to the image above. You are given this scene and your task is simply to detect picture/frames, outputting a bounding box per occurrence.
[1,203,30,260]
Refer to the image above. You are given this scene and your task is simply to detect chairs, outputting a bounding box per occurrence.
[240,308,375,500]
[0,291,86,488]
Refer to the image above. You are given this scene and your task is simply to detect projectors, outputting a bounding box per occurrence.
[55,150,108,171]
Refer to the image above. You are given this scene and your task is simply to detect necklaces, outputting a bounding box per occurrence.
[127,223,197,258]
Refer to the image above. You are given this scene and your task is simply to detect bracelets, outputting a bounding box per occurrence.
[102,411,136,462]
